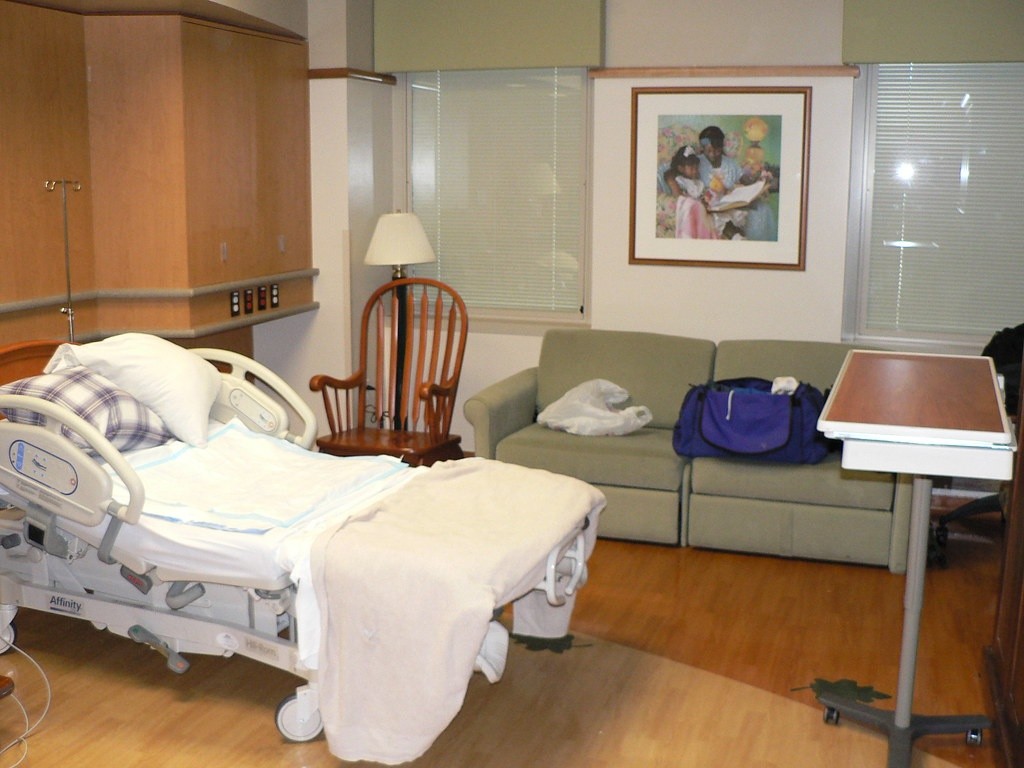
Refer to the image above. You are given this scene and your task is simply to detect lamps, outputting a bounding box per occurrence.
[365,210,436,432]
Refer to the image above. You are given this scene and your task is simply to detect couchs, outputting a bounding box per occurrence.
[463,328,911,574]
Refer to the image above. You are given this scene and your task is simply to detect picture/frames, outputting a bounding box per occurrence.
[629,86,811,270]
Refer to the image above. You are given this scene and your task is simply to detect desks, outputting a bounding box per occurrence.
[816,349,1019,767]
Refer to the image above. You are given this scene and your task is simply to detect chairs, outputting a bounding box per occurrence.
[309,278,468,467]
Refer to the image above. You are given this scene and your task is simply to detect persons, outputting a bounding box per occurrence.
[666,146,719,241]
[665,126,754,241]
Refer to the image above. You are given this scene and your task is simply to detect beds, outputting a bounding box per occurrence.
[0,347,606,765]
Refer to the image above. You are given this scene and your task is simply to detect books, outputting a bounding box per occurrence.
[706,180,770,213]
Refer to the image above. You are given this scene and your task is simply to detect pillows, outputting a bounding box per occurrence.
[44,333,220,448]
[0,364,174,458]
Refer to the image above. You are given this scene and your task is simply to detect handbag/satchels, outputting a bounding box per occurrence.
[673,377,828,464]
